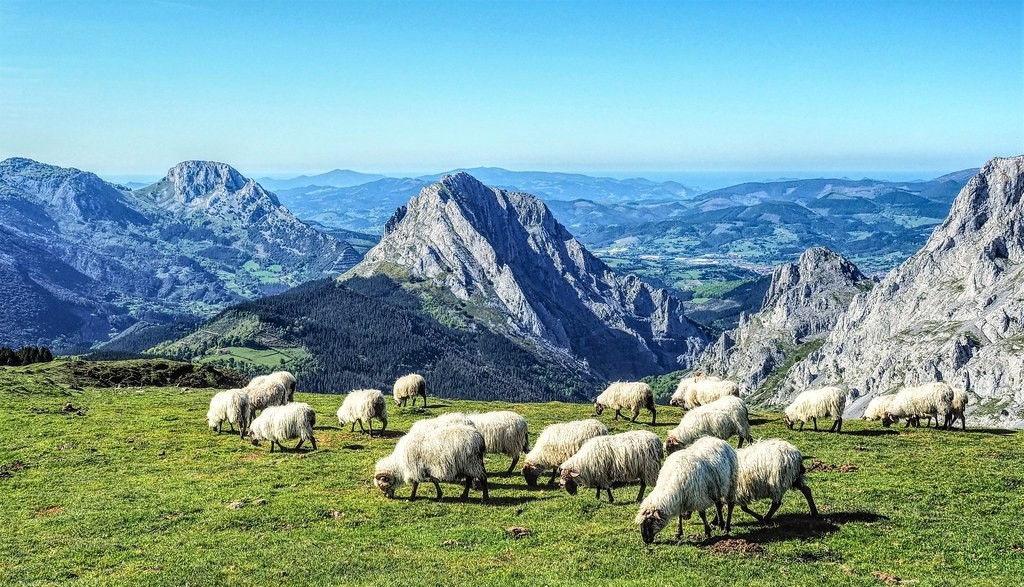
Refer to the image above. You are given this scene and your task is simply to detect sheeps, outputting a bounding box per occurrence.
[393,373,428,409]
[373,409,531,506]
[207,370,319,453]
[783,386,847,433]
[336,389,388,438]
[863,381,968,431]
[520,373,821,546]
